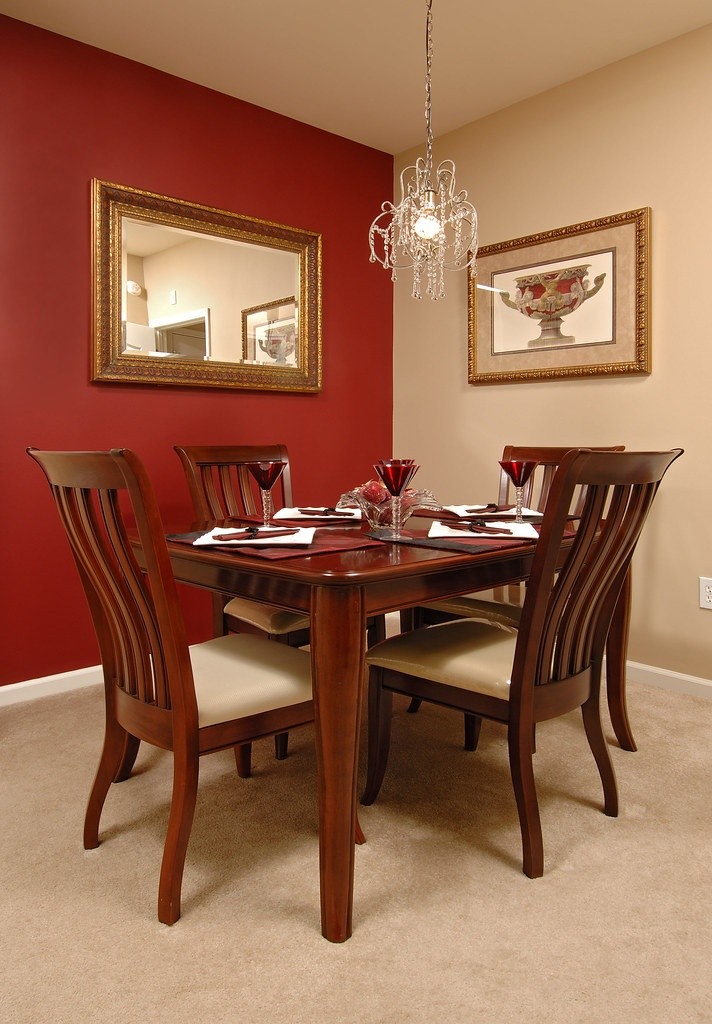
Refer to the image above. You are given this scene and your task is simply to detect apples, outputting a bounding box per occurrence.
[361,480,408,505]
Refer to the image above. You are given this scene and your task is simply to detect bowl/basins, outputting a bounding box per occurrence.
[343,481,441,529]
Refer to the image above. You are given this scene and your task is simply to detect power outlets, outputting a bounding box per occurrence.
[699,577,712,610]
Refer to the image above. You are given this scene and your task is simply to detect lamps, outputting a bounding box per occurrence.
[365,0,481,298]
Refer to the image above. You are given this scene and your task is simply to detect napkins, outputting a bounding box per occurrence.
[442,504,545,518]
[272,507,361,520]
[192,526,317,546]
[427,520,539,539]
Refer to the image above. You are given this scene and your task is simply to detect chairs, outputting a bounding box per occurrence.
[362,445,684,879]
[175,444,387,778]
[26,448,366,926]
[399,446,626,751]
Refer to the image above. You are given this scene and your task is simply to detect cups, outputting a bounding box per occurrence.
[377,458,415,464]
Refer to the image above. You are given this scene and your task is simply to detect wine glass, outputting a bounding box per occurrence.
[373,464,421,541]
[243,461,287,528]
[499,264,605,346]
[498,460,541,524]
[258,323,295,364]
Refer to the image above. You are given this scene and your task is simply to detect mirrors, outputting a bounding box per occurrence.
[90,178,321,391]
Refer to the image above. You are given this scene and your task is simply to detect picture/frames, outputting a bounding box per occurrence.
[466,207,652,384]
[241,296,295,362]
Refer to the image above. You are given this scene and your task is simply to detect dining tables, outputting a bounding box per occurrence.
[123,504,638,942]
